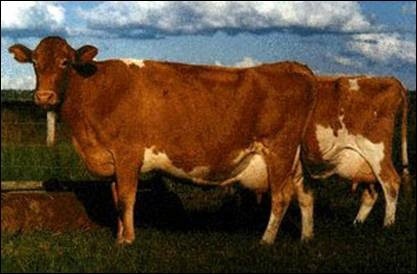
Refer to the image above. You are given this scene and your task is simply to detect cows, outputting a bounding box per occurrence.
[7,34,337,247]
[300,75,411,232]
